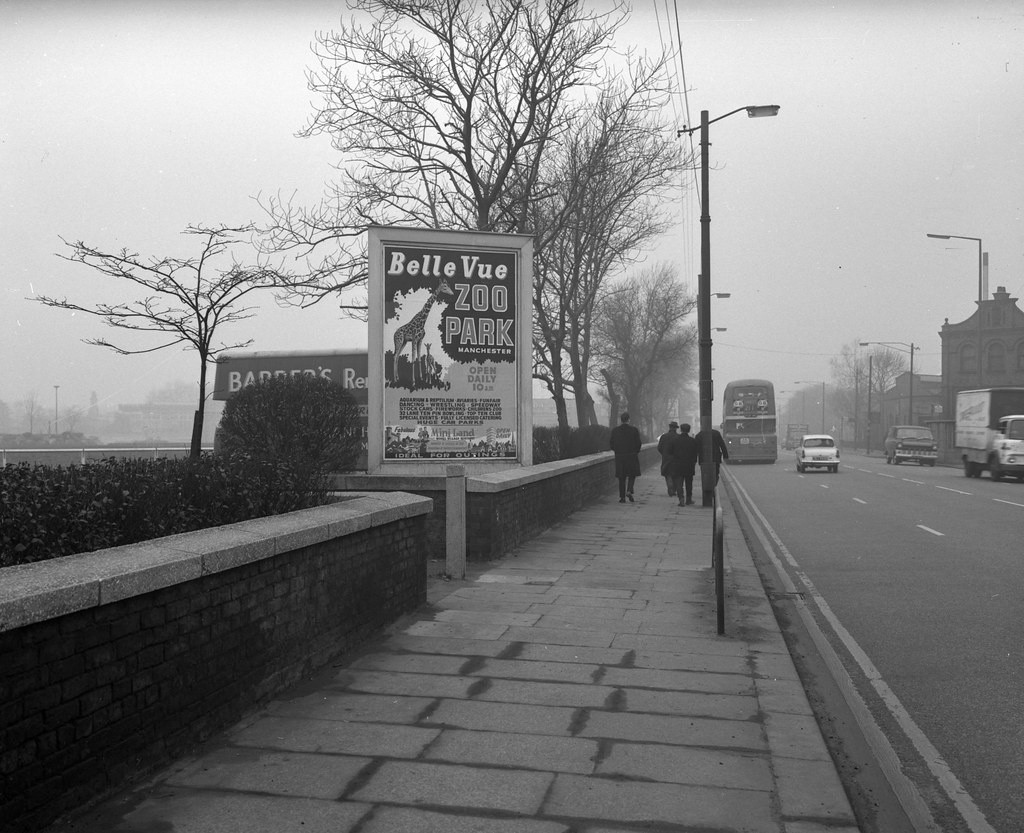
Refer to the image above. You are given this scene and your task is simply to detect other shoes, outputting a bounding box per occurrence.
[677,502,686,507]
[686,500,696,505]
[619,498,626,503]
[626,492,634,502]
[668,491,676,497]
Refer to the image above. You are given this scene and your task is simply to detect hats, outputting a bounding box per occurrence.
[669,422,679,429]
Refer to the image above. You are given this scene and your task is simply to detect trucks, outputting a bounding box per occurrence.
[953,386,1024,484]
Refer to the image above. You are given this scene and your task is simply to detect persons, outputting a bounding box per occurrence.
[610,411,642,502]
[656,421,684,496]
[692,420,730,501]
[677,423,698,508]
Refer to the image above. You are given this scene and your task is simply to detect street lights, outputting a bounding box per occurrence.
[780,390,805,423]
[927,232,984,387]
[677,102,783,504]
[793,380,826,434]
[858,341,921,425]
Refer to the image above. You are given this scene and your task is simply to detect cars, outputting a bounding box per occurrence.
[884,425,939,467]
[794,434,841,473]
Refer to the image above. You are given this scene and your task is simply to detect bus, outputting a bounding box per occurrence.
[719,378,778,465]
